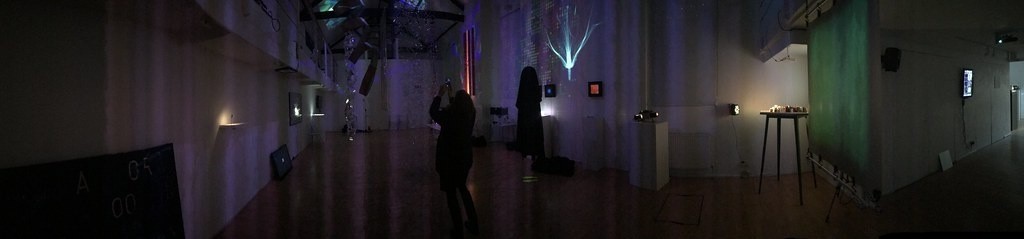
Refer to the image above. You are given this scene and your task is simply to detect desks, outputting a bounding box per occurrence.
[758,111,818,206]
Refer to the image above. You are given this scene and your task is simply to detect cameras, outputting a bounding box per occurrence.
[444,76,452,87]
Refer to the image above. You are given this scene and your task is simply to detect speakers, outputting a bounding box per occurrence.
[882,47,902,72]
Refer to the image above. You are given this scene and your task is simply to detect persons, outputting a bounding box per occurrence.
[514,67,545,161]
[428,83,480,239]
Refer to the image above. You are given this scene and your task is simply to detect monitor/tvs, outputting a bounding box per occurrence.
[962,69,973,98]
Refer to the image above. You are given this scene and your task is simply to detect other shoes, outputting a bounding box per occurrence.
[464,220,479,230]
[450,230,464,239]
